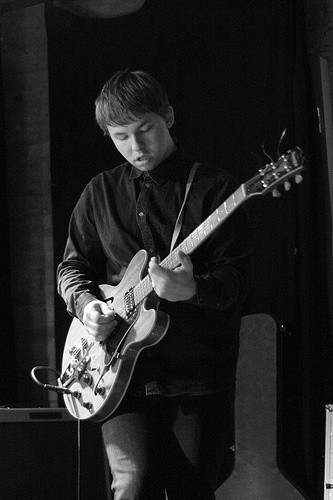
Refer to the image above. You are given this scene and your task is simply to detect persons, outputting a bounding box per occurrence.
[56,68,256,500]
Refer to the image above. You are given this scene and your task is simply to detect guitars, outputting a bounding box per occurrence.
[31,145,306,423]
